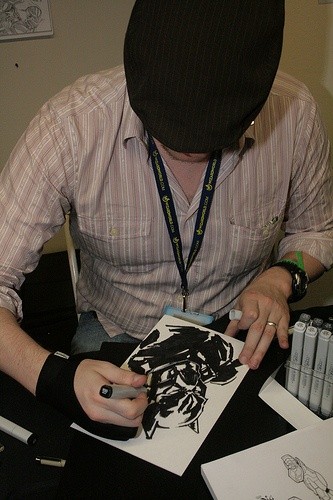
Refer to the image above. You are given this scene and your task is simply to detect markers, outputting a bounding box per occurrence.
[99,384,148,404]
[285,310,333,418]
[0,413,38,447]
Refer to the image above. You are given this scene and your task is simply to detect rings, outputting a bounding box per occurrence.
[266,321,278,329]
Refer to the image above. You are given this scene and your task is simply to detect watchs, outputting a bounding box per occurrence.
[269,260,310,304]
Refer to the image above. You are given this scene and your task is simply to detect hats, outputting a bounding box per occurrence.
[124,0,286,153]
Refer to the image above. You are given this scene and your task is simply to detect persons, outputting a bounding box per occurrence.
[0,0,333,426]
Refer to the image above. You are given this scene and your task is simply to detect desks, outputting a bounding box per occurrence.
[0,304,333,500]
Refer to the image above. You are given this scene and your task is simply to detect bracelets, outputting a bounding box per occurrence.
[271,250,305,272]
